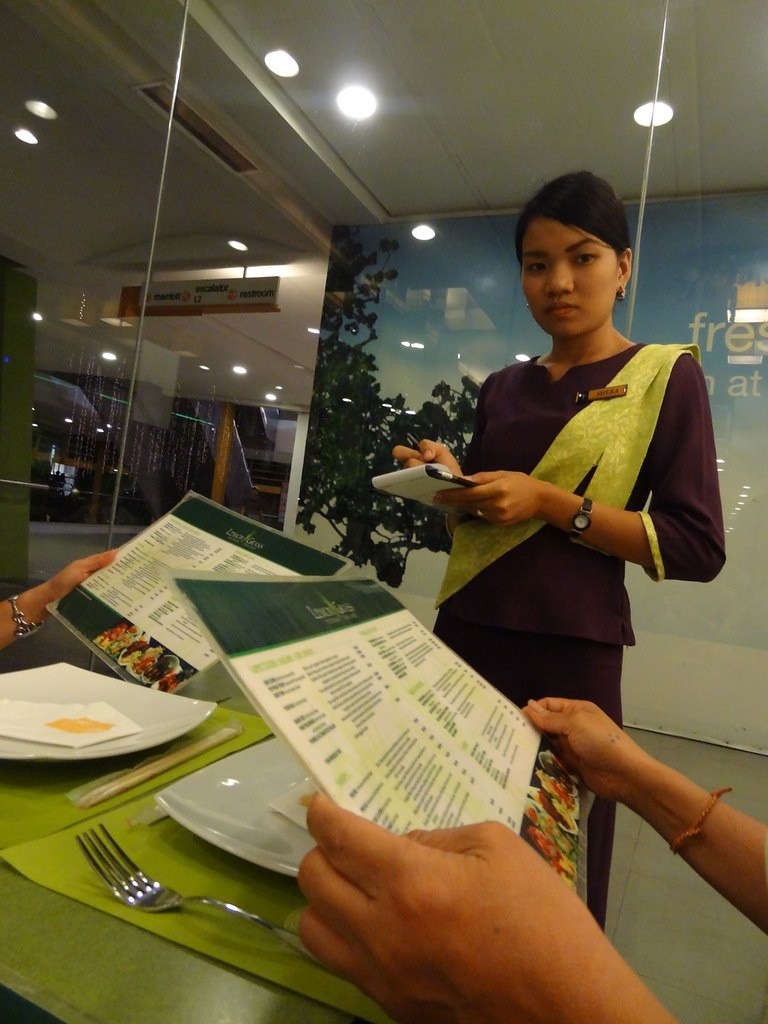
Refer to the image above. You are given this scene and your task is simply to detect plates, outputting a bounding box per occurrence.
[117,634,150,666]
[0,662,217,762]
[156,738,317,877]
[141,654,181,685]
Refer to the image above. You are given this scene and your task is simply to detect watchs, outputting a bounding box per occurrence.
[568,496,594,546]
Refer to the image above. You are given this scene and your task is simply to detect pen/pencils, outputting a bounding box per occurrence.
[405,432,438,464]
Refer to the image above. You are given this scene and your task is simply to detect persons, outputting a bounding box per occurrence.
[0,548,117,652]
[390,171,728,936]
[297,695,768,1024]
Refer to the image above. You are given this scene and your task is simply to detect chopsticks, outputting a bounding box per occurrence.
[81,728,238,806]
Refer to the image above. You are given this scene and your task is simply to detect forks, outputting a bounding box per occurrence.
[76,822,330,968]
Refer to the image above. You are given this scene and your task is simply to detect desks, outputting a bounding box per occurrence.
[0,661,611,1023]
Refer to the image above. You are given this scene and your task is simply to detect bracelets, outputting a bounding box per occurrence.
[669,786,734,855]
[7,593,45,639]
